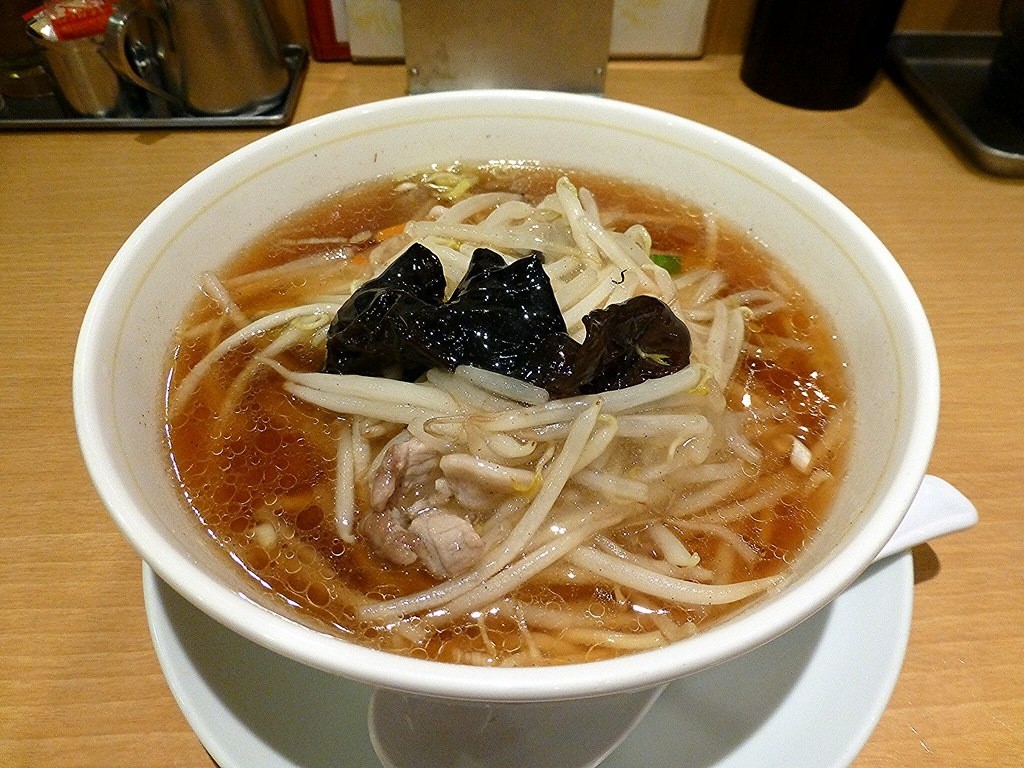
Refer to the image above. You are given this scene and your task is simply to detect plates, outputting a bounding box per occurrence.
[140,549,915,768]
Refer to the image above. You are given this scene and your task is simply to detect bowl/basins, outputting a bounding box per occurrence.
[70,88,940,702]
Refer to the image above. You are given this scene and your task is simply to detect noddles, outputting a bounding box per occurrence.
[165,165,853,666]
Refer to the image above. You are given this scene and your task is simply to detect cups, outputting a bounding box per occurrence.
[102,0,292,118]
[22,2,156,117]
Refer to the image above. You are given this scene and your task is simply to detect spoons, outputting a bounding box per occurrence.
[368,471,981,768]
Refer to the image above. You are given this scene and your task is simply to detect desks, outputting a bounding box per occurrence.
[0,53,1024,768]
